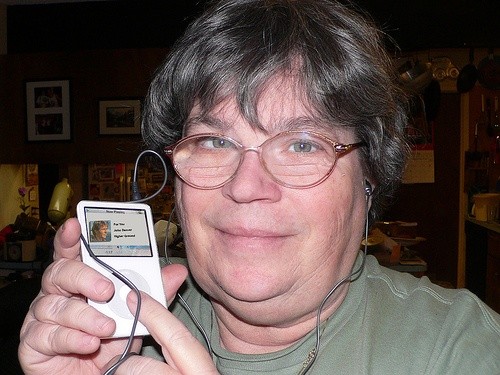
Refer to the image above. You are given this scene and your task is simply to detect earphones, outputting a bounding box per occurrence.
[365,180,372,196]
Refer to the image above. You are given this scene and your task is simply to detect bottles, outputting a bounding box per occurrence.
[47,178,73,224]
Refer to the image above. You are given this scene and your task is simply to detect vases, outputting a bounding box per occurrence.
[6,233,37,262]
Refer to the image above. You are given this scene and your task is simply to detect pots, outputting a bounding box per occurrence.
[400,62,433,91]
[479,45,500,90]
[457,46,478,94]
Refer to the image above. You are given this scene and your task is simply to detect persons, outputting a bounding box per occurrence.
[17,0,500,375]
[91,221,109,241]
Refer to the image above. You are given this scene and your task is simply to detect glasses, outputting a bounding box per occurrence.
[163,132,365,189]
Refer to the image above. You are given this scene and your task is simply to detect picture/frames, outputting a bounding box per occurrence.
[23,79,73,143]
[94,95,144,138]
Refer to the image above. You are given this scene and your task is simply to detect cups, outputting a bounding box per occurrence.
[472,192,500,221]
[485,199,500,224]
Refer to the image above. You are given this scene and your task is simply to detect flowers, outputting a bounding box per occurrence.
[9,187,39,231]
